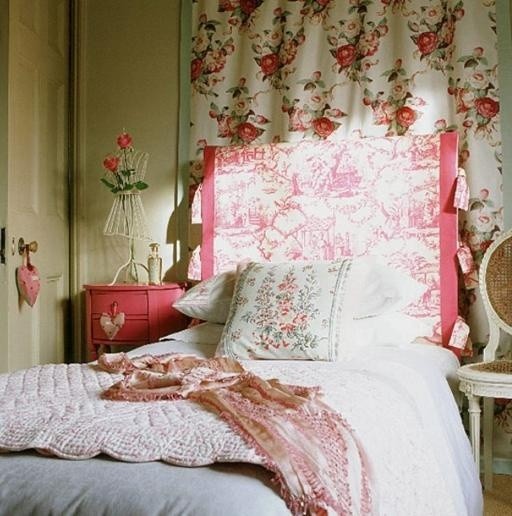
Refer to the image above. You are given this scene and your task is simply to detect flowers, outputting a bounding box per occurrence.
[100,131,148,233]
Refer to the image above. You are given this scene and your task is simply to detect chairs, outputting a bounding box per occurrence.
[458,224,511,494]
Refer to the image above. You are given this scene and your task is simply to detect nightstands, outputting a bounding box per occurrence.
[83,282,193,360]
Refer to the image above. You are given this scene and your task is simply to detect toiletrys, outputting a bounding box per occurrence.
[148,243,162,284]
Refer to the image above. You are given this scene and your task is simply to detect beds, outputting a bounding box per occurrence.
[0,132,469,516]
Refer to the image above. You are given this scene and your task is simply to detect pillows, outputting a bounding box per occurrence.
[172,257,430,361]
